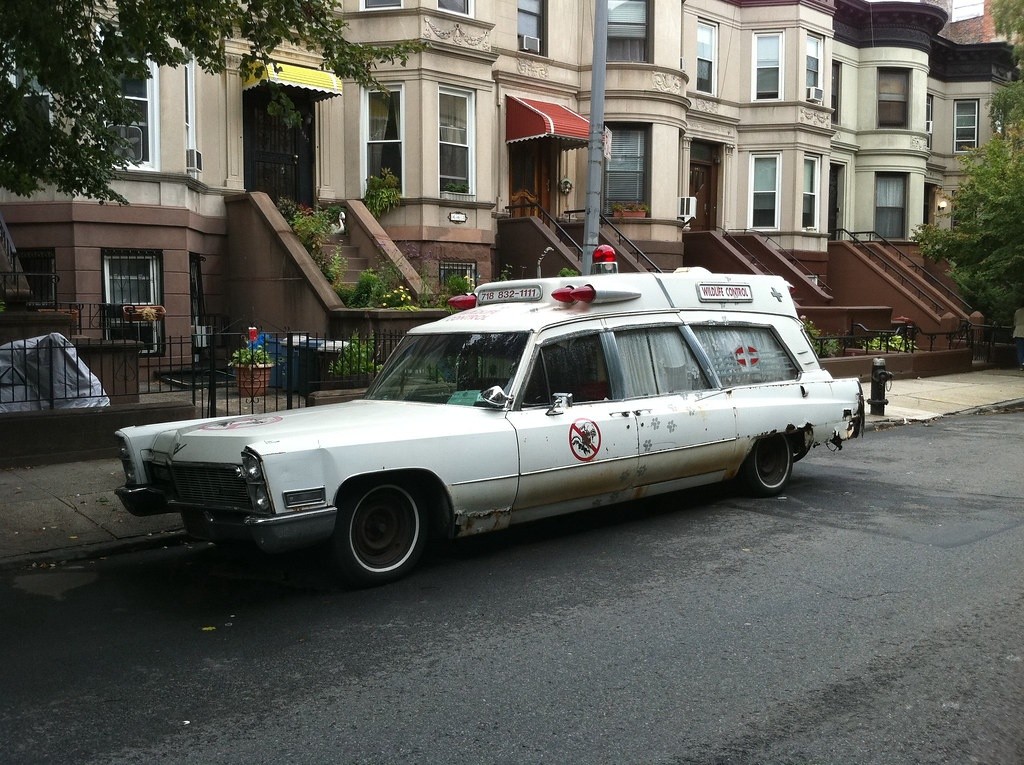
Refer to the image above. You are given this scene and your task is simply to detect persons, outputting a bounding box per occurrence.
[1013,298,1024,371]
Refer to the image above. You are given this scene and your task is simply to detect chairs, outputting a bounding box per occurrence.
[541,344,577,394]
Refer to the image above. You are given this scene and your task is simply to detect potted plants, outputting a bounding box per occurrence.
[333,283,355,302]
[440,182,476,202]
[328,328,381,389]
[225,335,275,397]
[123,304,166,323]
[612,200,651,226]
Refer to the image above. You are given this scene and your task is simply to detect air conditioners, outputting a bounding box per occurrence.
[926,121,933,134]
[519,34,540,54]
[185,149,202,173]
[191,325,207,348]
[806,87,824,102]
[678,197,697,218]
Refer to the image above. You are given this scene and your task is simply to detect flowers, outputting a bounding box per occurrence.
[381,286,412,308]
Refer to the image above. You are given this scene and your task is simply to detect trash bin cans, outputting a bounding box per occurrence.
[268,334,350,394]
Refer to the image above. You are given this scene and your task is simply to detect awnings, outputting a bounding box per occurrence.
[506,94,591,152]
[241,53,343,104]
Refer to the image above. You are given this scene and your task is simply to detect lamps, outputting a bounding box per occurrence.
[304,112,313,127]
[938,201,947,211]
[714,152,720,164]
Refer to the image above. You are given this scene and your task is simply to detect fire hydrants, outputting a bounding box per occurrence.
[867,357,895,416]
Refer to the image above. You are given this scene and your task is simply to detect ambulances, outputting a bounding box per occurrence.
[115,245,867,598]
